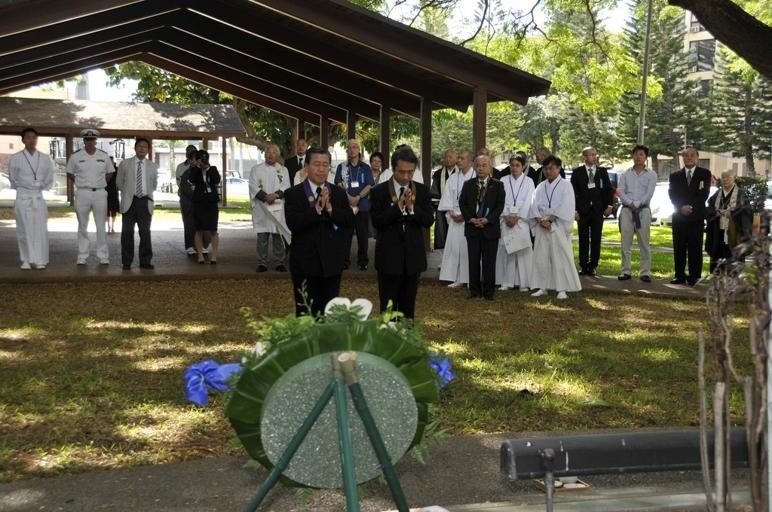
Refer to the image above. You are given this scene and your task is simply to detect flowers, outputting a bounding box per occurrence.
[182,278,456,507]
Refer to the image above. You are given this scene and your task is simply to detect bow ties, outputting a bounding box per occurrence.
[76,258,87,265]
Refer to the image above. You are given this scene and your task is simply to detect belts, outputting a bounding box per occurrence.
[622,204,650,208]
[77,187,105,192]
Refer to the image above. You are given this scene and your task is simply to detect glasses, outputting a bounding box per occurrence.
[583,153,598,157]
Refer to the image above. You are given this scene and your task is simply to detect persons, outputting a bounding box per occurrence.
[181,150,211,255]
[187,149,221,264]
[668,148,711,286]
[8,128,54,270]
[618,144,657,282]
[705,169,754,277]
[115,138,157,270]
[105,157,120,233]
[67,129,115,265]
[250,138,613,329]
[176,145,197,198]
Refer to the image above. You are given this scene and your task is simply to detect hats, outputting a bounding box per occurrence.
[79,128,100,140]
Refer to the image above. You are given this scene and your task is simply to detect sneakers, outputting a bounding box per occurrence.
[275,264,287,272]
[185,247,197,256]
[256,266,268,272]
[520,288,529,292]
[343,262,349,270]
[32,263,46,270]
[498,286,508,291]
[201,247,209,254]
[19,262,32,270]
[557,291,568,300]
[101,258,110,265]
[531,289,548,296]
[448,282,463,289]
[358,263,368,271]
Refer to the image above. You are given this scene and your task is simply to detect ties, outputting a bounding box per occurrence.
[316,187,330,217]
[479,180,485,211]
[136,161,143,199]
[686,170,692,187]
[399,187,409,216]
[588,167,594,184]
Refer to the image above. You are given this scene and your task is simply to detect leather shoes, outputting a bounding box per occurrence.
[123,264,130,270]
[640,276,651,283]
[671,277,686,284]
[579,268,587,275]
[140,263,154,270]
[588,268,597,276]
[618,274,631,280]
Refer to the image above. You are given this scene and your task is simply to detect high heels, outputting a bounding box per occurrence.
[210,256,216,264]
[197,256,205,264]
